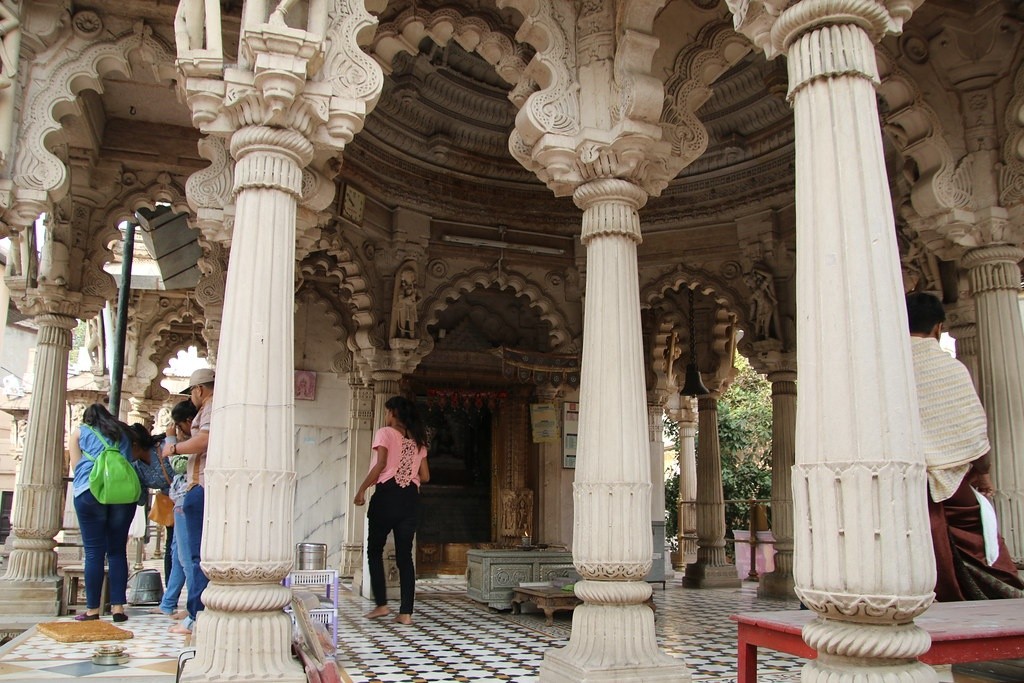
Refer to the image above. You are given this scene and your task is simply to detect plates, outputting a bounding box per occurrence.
[516,545,537,551]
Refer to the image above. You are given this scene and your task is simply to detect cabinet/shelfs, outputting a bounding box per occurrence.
[285,569,343,649]
[645,521,667,589]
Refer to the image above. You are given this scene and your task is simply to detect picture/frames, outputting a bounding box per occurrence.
[562,400,580,469]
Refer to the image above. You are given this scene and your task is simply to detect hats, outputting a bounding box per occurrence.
[179,368,216,395]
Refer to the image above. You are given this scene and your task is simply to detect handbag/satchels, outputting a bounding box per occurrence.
[149,491,174,526]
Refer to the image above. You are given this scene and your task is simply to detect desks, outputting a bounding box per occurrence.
[512,587,655,629]
[729,597,1024,683]
[732,529,774,580]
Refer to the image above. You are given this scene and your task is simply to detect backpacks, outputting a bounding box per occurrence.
[78,422,141,505]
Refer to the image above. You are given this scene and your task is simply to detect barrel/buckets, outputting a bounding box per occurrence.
[128,572,164,604]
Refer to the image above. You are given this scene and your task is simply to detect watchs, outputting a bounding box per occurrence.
[168,442,180,455]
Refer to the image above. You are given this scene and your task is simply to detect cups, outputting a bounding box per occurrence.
[522,536,531,546]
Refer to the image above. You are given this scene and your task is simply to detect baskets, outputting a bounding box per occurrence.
[284,570,338,625]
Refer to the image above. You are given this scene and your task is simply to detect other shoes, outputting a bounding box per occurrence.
[114,613,128,621]
[75,612,100,622]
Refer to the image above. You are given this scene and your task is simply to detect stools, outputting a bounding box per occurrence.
[61,565,111,617]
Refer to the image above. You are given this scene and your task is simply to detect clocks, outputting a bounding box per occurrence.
[336,178,367,228]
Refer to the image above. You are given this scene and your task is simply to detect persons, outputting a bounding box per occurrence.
[903,290,1024,602]
[151,397,193,620]
[128,422,179,608]
[68,403,138,623]
[353,395,430,625]
[161,367,216,634]
